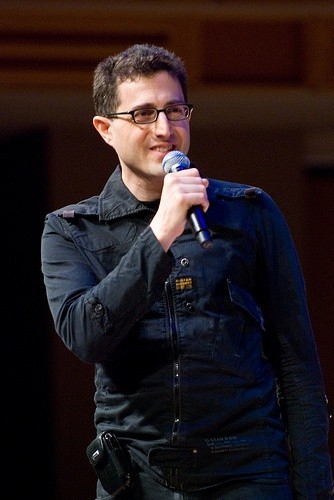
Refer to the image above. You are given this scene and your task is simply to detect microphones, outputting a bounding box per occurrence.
[162,150,213,251]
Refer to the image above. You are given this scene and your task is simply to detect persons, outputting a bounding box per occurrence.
[40,44,333,500]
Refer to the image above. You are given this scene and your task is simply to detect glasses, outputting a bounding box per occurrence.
[108,104,191,125]
